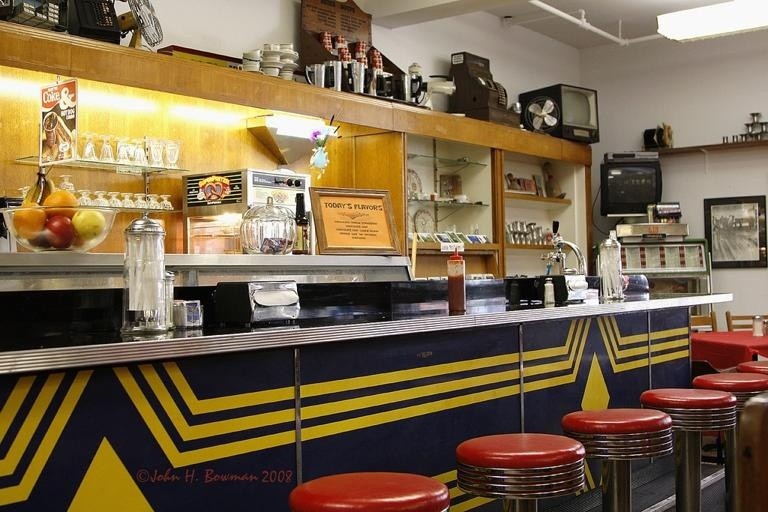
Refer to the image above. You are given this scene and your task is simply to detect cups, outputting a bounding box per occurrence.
[304,59,424,104]
[237,42,300,80]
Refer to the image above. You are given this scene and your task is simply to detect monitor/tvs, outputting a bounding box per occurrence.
[599,162,663,216]
[518,84,600,145]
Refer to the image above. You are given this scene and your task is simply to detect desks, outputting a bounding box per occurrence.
[691,330,767,374]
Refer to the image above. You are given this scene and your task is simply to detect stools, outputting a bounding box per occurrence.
[642,388,737,511]
[561,407,675,511]
[739,360,767,374]
[456,433,588,511]
[288,470,451,511]
[692,371,768,511]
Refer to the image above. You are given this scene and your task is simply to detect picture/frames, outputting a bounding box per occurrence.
[703,195,767,269]
[309,186,402,256]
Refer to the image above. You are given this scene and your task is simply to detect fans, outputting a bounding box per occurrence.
[116,0,164,49]
[525,95,561,136]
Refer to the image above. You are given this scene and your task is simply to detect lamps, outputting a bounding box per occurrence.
[655,1,768,44]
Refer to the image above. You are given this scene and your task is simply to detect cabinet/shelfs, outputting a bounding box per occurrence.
[0,20,595,287]
[17,154,192,220]
[593,239,713,333]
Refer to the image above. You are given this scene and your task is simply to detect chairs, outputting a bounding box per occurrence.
[690,311,718,333]
[726,311,768,331]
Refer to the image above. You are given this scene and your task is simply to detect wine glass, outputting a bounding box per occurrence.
[107,191,123,207]
[59,173,75,190]
[121,192,135,207]
[147,195,161,208]
[160,194,174,208]
[92,191,110,206]
[77,189,93,205]
[135,194,147,208]
[81,131,182,169]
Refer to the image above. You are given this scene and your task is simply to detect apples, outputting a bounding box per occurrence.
[72,209,106,242]
[42,216,74,250]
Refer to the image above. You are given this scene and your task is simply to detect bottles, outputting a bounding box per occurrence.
[599,228,623,299]
[543,277,557,306]
[293,192,311,255]
[122,217,167,333]
[446,247,467,313]
[163,269,175,333]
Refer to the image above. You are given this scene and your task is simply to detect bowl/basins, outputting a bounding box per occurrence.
[0,204,118,254]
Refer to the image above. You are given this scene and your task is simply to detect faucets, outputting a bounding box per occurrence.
[557,241,587,275]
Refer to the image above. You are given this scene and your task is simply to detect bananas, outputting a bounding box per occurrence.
[22,173,52,204]
